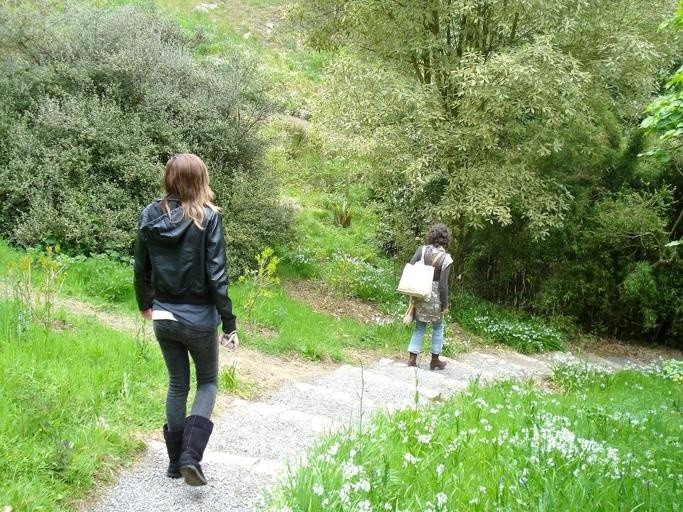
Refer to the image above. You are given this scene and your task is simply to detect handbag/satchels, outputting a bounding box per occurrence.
[402,301,415,325]
[397,246,435,299]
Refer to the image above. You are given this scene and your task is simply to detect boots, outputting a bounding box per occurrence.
[408,352,418,367]
[430,354,447,370]
[163,414,214,486]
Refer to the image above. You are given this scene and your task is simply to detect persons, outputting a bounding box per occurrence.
[403,221,454,372]
[132,151,239,486]
